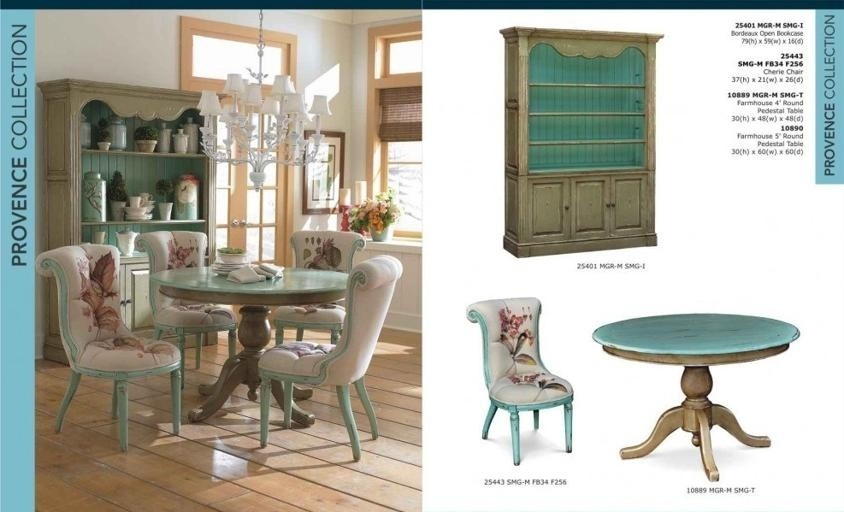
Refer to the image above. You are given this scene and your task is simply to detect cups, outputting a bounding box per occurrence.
[141,192,154,200]
[129,196,147,209]
[93,231,107,245]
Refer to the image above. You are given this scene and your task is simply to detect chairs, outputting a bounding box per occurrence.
[267,230,366,345]
[253,250,404,463]
[130,229,238,370]
[465,293,576,467]
[34,240,181,454]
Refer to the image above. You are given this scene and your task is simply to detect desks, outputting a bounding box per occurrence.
[591,312,800,483]
[149,264,351,428]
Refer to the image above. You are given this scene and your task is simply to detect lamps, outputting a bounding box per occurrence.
[194,10,333,194]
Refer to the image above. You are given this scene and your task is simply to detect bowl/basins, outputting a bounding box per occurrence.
[123,208,146,215]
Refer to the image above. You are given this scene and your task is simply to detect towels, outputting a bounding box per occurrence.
[209,260,285,287]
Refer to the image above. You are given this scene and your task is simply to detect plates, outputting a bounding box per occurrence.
[210,262,245,277]
[124,214,152,221]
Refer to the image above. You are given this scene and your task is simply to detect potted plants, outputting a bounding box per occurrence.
[216,243,246,264]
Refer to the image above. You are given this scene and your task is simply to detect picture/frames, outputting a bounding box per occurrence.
[301,129,345,217]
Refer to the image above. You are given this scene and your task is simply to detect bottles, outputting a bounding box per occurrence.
[158,116,200,155]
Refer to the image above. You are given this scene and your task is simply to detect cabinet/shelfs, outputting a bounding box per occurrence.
[36,77,217,370]
[498,27,666,260]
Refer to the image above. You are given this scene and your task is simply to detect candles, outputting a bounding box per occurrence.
[338,187,351,206]
[354,181,367,204]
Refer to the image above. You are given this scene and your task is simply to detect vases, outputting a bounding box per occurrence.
[369,222,388,242]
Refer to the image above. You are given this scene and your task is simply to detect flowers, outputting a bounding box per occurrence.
[348,187,402,231]
